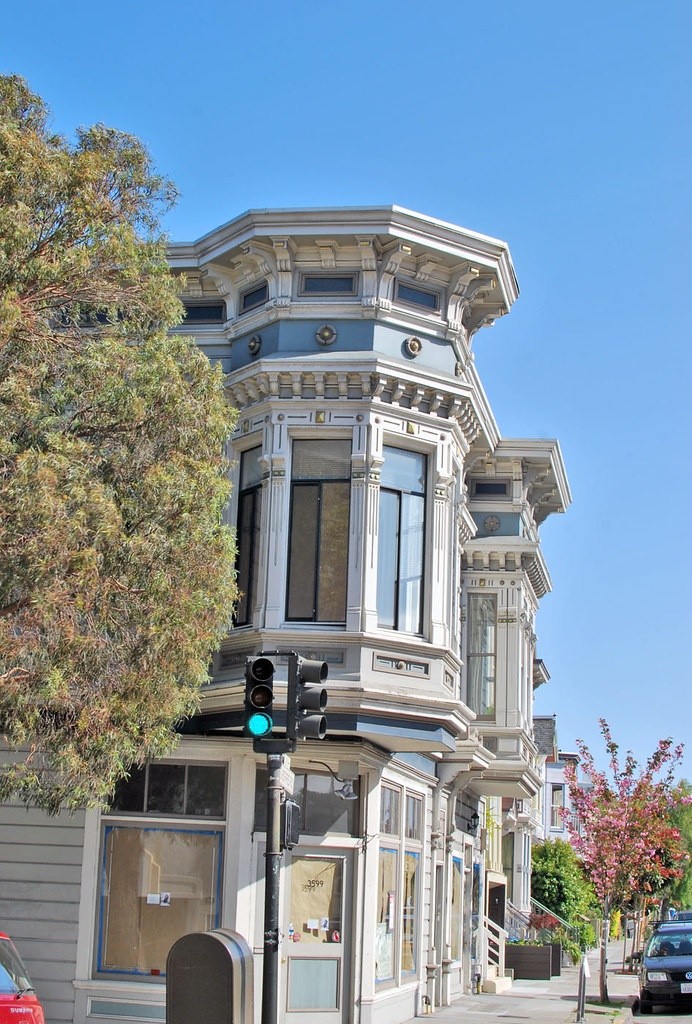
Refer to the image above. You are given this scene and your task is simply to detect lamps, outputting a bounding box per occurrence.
[467,812,480,832]
[312,756,359,802]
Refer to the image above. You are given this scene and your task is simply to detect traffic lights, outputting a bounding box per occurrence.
[242,655,276,739]
[286,655,329,743]
[280,798,302,851]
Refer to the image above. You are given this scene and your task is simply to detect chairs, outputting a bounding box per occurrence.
[660,941,675,955]
[679,941,689,955]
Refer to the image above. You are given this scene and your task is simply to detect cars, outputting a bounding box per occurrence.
[631,909,692,1014]
[0,929,45,1024]
